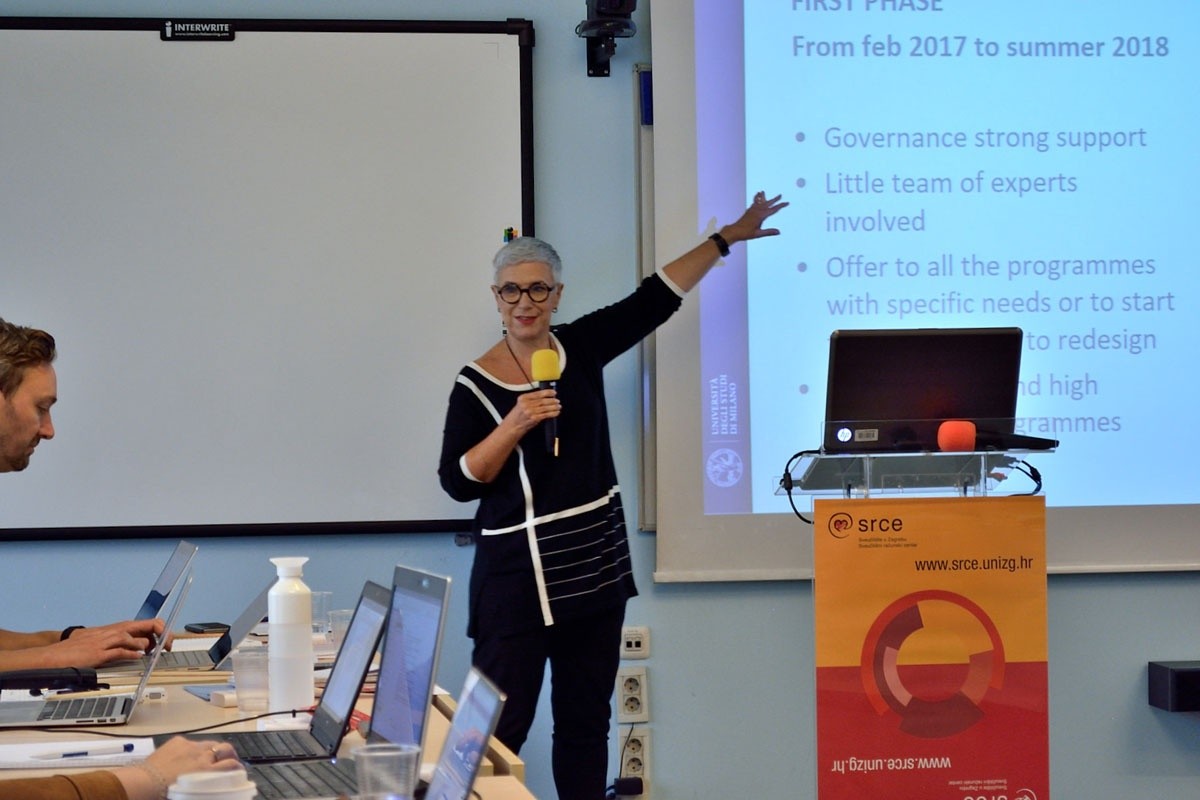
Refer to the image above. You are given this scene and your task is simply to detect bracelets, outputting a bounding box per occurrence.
[708,233,732,257]
[60,625,86,642]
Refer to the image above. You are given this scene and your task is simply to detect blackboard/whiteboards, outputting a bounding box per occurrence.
[0,15,539,545]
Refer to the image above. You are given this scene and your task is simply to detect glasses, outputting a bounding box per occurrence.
[495,280,557,305]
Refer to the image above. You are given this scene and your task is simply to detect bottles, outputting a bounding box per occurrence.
[266,557,314,710]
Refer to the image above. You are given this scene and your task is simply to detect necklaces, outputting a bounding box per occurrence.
[503,333,554,391]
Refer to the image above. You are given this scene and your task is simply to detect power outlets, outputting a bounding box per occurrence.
[616,625,654,800]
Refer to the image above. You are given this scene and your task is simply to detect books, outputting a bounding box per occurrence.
[312,633,379,693]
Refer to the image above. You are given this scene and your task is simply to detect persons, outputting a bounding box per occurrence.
[0,319,174,673]
[0,735,242,800]
[434,189,791,800]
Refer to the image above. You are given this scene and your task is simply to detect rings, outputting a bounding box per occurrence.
[209,747,219,762]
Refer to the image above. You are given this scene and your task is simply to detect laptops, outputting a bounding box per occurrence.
[0,567,196,729]
[423,665,507,800]
[152,577,394,765]
[244,564,452,799]
[799,326,1023,490]
[93,575,279,682]
[130,536,199,622]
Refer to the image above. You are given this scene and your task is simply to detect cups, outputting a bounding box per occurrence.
[312,592,355,654]
[351,742,420,800]
[231,651,269,711]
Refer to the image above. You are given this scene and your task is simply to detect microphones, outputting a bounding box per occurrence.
[530,348,562,460]
[935,418,1062,452]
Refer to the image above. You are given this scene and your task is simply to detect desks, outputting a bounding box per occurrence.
[0,627,544,799]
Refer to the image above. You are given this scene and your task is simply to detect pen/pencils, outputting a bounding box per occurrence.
[30,743,134,760]
